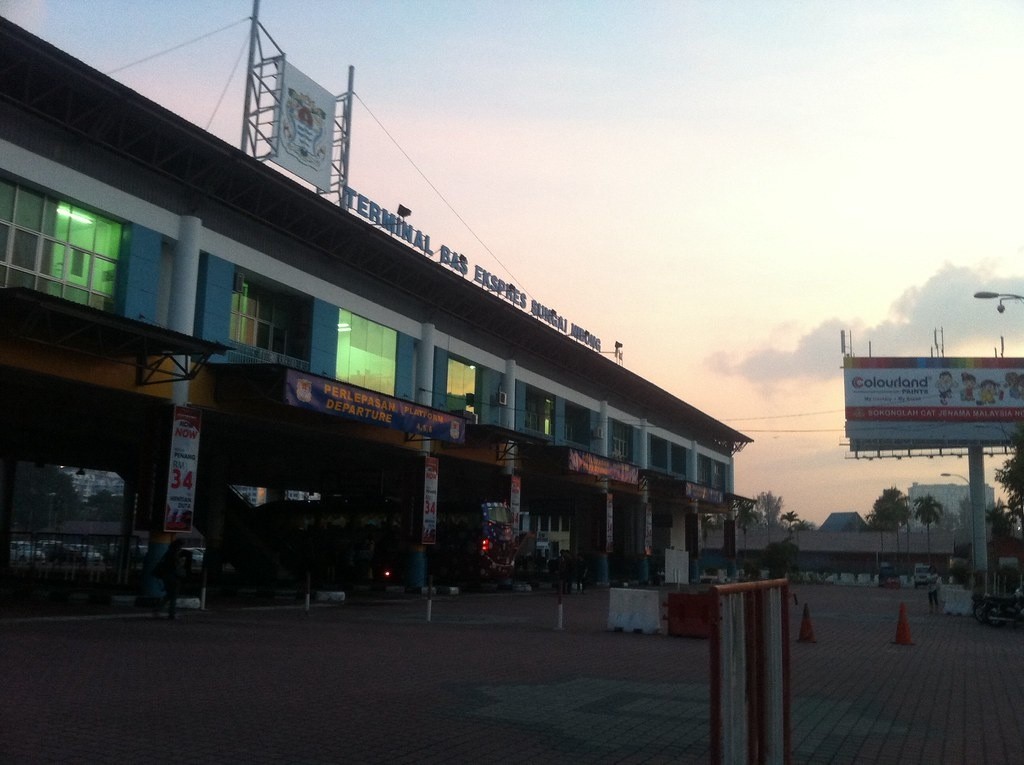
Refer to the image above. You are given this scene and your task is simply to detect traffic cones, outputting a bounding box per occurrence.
[796,604,818,644]
[890,602,915,645]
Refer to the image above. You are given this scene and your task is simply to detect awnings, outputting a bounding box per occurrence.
[465,424,550,461]
[547,445,640,486]
[208,363,471,443]
[0,285,236,386]
[643,468,723,506]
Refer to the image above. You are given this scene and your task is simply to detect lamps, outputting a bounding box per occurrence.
[396,204,412,218]
[459,254,623,350]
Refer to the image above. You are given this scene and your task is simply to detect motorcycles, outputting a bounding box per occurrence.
[974,592,1024,627]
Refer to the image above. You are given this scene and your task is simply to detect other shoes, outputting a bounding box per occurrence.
[151,603,180,622]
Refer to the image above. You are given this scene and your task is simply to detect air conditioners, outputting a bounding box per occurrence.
[593,427,603,438]
[233,272,244,292]
[495,392,506,404]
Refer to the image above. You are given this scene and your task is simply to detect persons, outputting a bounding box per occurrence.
[926,566,938,614]
[152,538,186,621]
[558,549,587,594]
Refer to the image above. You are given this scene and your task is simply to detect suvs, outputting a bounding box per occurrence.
[880,567,901,589]
[915,565,931,587]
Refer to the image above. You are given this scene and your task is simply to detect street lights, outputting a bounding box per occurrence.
[975,293,1024,300]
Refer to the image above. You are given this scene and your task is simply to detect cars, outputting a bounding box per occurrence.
[9,541,206,568]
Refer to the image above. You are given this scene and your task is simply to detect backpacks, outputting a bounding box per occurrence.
[149,560,177,579]
